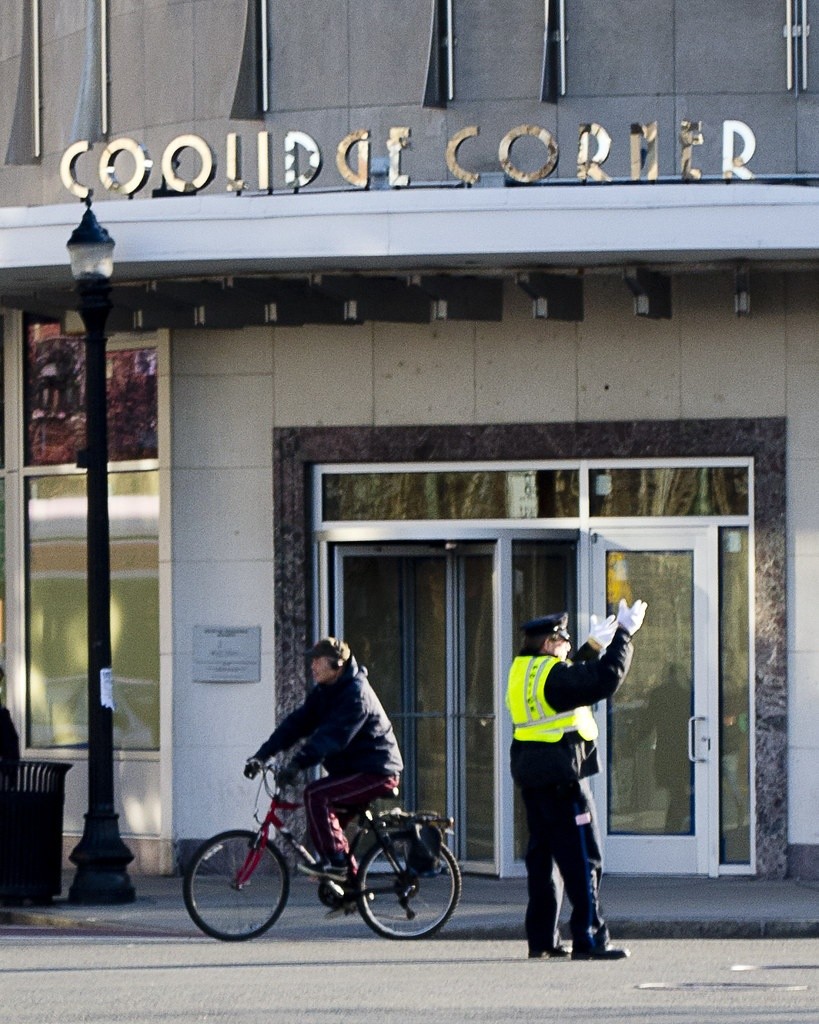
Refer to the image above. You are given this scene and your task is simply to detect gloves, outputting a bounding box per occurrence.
[618,597,648,636]
[590,614,620,649]
[274,768,292,791]
[244,761,260,780]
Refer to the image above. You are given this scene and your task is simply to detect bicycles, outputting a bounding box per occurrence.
[182,758,463,941]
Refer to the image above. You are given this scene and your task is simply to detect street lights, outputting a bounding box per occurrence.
[65,195,140,905]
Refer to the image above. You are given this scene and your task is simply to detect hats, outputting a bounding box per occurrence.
[302,637,350,661]
[527,612,570,640]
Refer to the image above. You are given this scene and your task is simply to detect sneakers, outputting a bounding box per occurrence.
[324,891,375,919]
[297,857,348,880]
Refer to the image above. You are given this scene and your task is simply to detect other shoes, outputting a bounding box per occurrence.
[571,947,626,960]
[529,943,570,958]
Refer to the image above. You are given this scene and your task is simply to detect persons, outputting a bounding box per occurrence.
[348,637,389,695]
[0,668,21,805]
[720,646,748,833]
[505,598,647,960]
[638,661,692,832]
[244,636,403,919]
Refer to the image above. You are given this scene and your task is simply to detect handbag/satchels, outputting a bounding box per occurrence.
[407,820,441,877]
